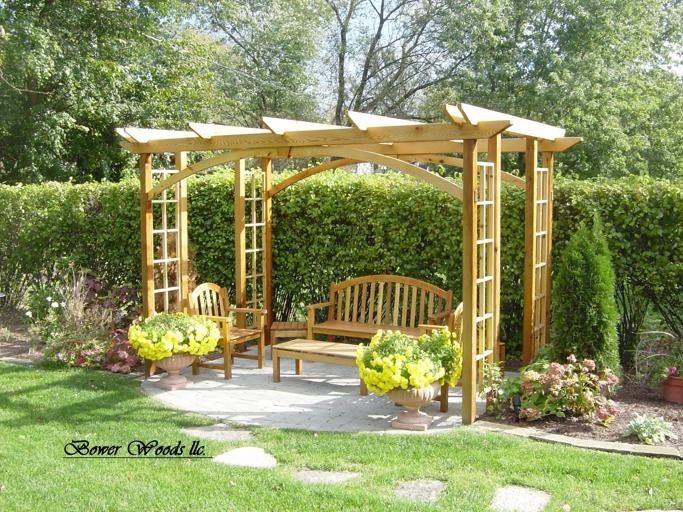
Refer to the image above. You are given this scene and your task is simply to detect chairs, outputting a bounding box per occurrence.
[395,301,463,412]
[187,283,267,380]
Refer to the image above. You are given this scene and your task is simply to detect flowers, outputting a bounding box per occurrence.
[641,341,683,388]
[127,312,221,361]
[355,328,463,397]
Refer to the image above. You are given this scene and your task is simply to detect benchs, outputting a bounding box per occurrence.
[307,275,453,342]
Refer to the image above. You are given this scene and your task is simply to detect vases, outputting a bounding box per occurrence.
[152,353,197,391]
[385,380,441,430]
[663,376,683,404]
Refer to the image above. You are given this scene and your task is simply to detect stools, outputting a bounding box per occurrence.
[478,341,505,382]
[270,322,307,360]
